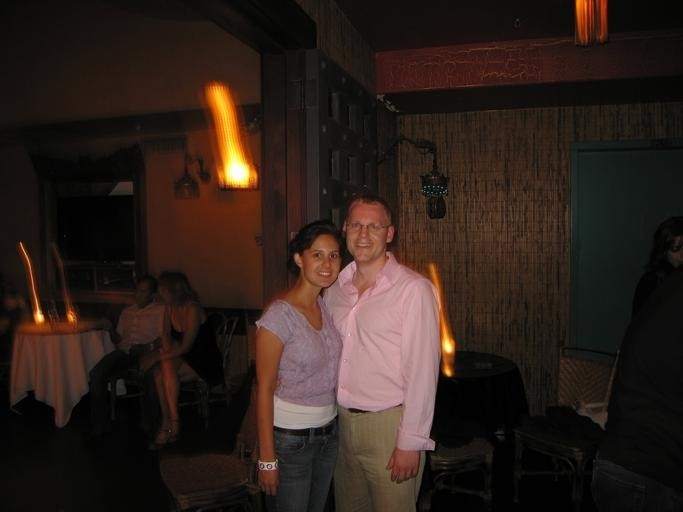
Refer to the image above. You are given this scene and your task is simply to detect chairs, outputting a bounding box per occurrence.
[520,342,620,510]
[111,372,144,420]
[418,438,494,512]
[158,374,265,512]
[179,310,238,432]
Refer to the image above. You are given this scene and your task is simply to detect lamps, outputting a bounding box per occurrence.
[163,135,211,201]
[574,1,608,50]
[376,135,449,218]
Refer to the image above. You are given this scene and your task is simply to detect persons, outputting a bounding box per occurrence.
[252,218,344,512]
[144,270,225,446]
[590,264,683,512]
[87,272,165,450]
[632,215,683,318]
[321,195,442,512]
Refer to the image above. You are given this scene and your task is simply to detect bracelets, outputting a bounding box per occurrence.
[257,458,281,473]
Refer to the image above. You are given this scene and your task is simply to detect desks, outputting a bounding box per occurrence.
[10,316,119,429]
[431,351,528,477]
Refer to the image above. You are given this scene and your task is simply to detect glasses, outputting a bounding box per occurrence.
[347,222,389,232]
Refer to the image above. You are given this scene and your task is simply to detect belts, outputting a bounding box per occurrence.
[346,408,370,413]
[273,426,332,435]
